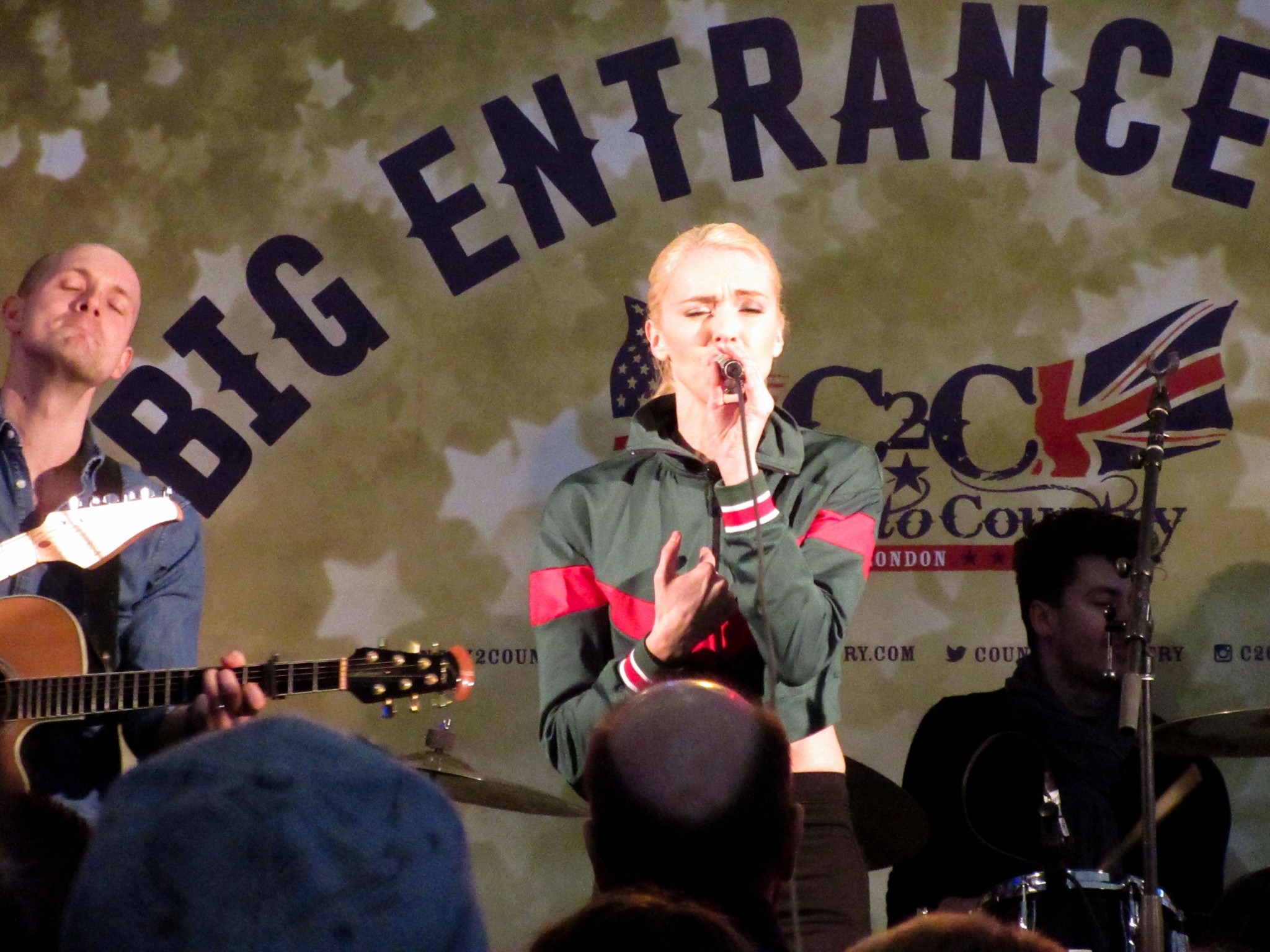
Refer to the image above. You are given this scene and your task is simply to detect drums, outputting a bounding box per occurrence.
[985,870,1192,952]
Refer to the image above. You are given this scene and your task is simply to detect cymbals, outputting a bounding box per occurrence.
[840,755,919,873]
[387,751,590,821]
[1152,706,1270,761]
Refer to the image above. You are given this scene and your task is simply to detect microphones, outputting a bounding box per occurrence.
[714,352,743,379]
[1042,771,1070,847]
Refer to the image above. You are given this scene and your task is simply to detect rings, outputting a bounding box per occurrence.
[214,703,225,711]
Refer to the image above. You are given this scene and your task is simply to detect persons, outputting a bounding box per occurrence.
[1,242,489,952]
[530,221,886,952]
[886,506,1230,952]
[527,679,1075,952]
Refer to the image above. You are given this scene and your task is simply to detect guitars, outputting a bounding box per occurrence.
[0,484,186,585]
[0,594,475,801]
